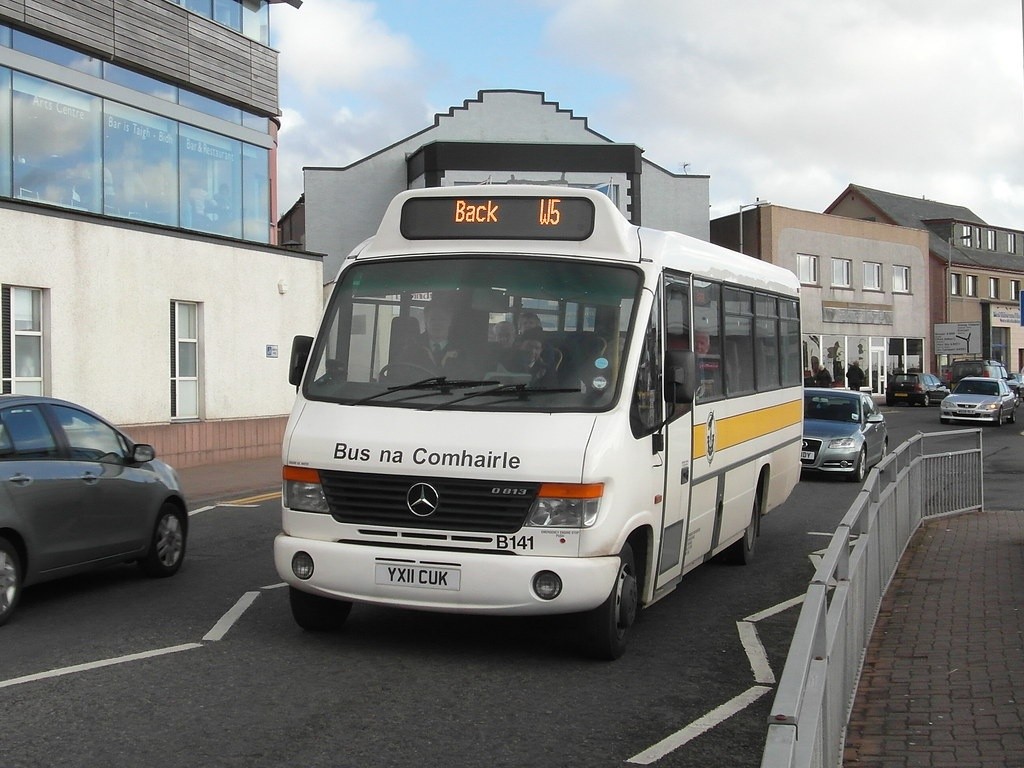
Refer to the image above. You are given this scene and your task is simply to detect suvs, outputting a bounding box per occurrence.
[945,356,1020,407]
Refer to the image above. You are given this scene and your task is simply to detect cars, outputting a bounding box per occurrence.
[885,373,951,407]
[1009,373,1024,401]
[939,376,1018,426]
[802,387,890,483]
[0,394,190,626]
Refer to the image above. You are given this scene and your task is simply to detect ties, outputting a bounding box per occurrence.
[433,343,441,361]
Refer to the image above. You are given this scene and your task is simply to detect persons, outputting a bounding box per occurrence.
[389,299,485,385]
[495,314,549,388]
[846,357,865,391]
[693,329,710,355]
[814,362,832,387]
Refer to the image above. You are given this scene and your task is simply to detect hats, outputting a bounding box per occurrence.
[521,325,545,343]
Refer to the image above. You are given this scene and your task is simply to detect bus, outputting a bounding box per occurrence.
[272,182,805,664]
